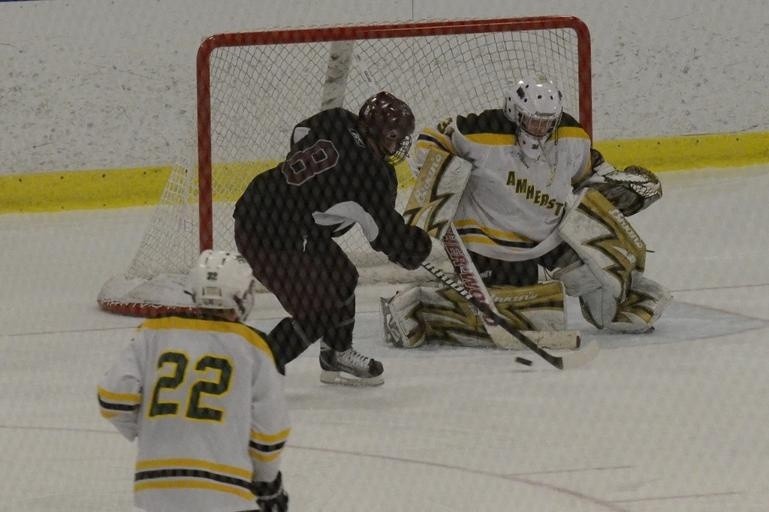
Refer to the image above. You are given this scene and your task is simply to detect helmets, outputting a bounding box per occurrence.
[190,248,256,323]
[359,90,416,147]
[504,74,565,160]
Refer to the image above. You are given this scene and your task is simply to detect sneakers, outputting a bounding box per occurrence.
[318,340,384,379]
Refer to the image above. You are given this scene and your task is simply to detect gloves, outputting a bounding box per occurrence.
[250,470,290,512]
[392,225,433,271]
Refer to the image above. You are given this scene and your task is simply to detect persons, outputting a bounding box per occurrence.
[98,250,289,512]
[389,74,673,347]
[235,91,433,379]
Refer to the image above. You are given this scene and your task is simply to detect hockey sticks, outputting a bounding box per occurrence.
[440,221,581,349]
[415,258,600,370]
